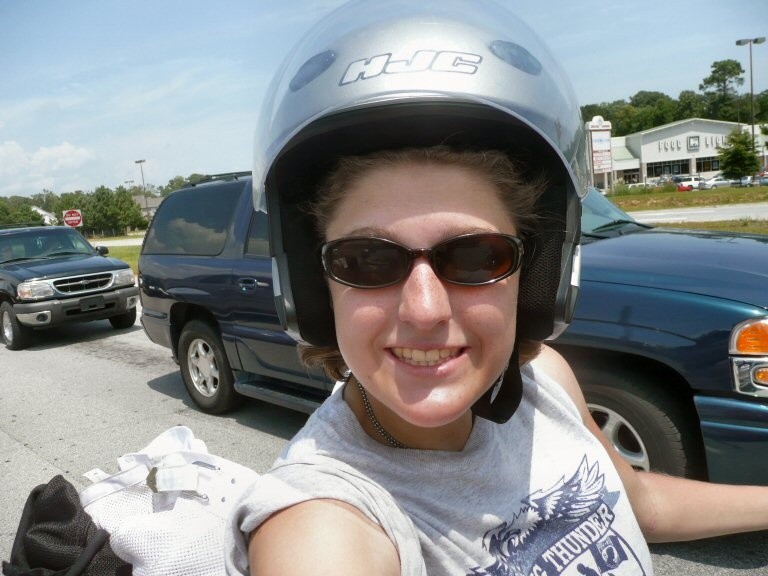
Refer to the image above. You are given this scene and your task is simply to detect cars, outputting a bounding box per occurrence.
[621,172,768,190]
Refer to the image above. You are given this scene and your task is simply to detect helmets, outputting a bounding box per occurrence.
[252,0,596,346]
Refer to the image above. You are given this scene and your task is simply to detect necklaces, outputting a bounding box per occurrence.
[357,382,405,448]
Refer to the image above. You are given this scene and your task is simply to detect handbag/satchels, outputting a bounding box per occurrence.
[79,425,261,576]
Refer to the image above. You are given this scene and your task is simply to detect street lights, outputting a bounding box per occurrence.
[735,38,766,156]
[135,159,151,220]
[123,180,135,198]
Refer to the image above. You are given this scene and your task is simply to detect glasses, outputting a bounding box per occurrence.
[318,232,529,287]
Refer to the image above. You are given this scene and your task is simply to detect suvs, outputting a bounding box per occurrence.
[137,170,768,489]
[0,224,141,351]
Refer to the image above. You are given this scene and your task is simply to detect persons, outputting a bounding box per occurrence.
[0,244,19,262]
[232,0,768,576]
[42,238,61,253]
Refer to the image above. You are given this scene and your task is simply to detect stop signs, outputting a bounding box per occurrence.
[63,210,82,226]
